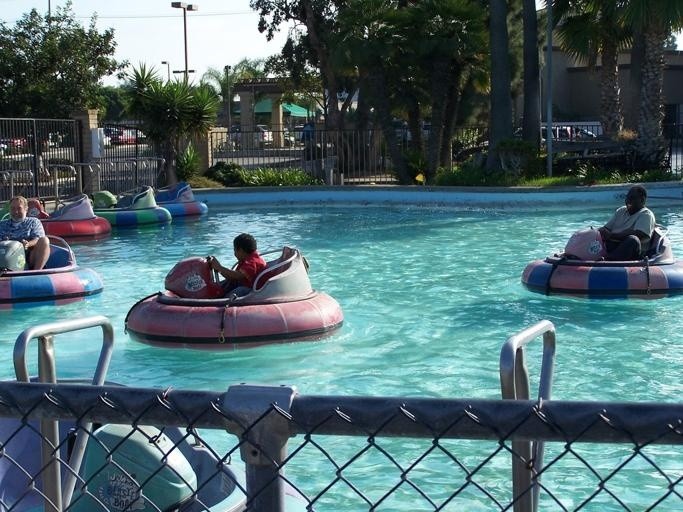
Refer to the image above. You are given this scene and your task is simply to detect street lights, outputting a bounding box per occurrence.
[161,61,170,83]
[225,64,233,142]
[173,70,195,84]
[169,2,199,86]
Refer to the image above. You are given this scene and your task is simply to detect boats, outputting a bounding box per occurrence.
[157,181,210,218]
[520,219,683,302]
[0,230,106,311]
[91,184,173,228]
[122,245,346,353]
[25,194,111,242]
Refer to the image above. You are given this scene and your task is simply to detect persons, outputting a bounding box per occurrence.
[599,186,655,260]
[209,233,270,299]
[0,196,50,270]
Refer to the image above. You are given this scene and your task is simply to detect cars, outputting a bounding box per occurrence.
[229,122,304,148]
[456,124,597,162]
[0,126,150,156]
